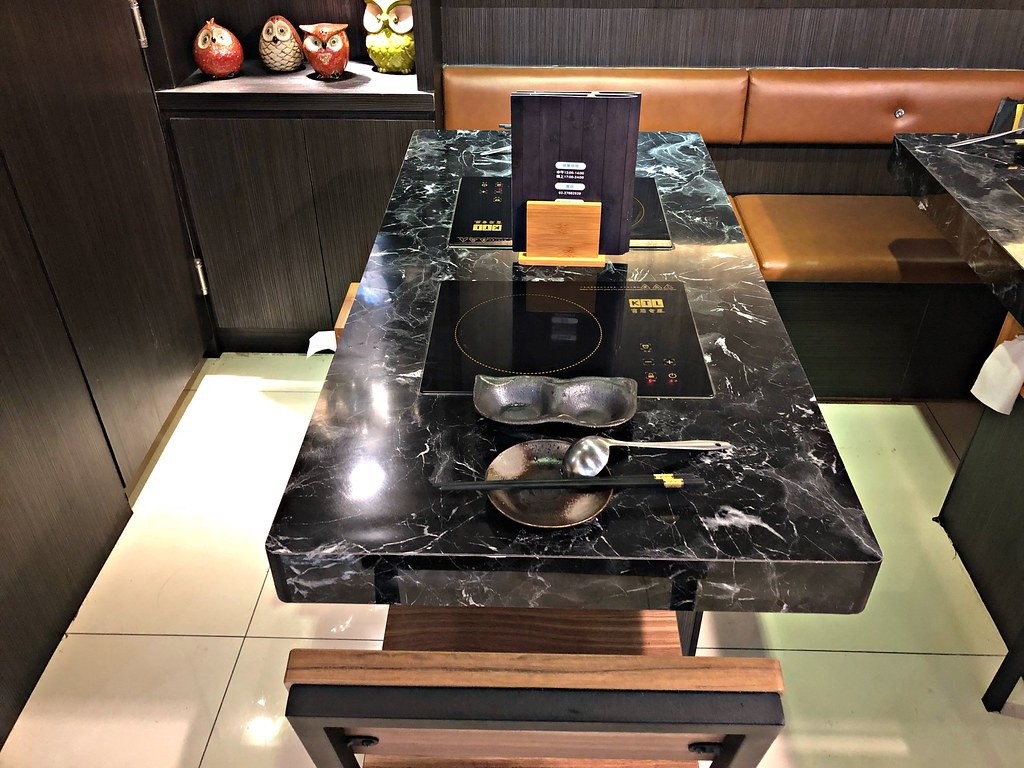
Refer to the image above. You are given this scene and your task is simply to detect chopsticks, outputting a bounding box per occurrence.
[439,474,704,490]
[1003,138,1016,146]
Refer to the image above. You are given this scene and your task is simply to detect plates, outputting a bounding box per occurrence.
[473,375,638,427]
[486,439,613,528]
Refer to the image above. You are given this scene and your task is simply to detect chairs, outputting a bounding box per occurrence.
[284,604,786,768]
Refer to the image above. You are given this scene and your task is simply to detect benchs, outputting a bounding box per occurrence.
[442,66,1023,405]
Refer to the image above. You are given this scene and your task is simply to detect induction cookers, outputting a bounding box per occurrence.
[448,176,674,248]
[417,279,715,400]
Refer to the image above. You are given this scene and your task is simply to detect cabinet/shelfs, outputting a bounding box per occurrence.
[165,116,415,328]
[0,0,206,750]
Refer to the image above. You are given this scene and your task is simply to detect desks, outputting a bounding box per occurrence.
[263,131,882,655]
[888,134,1024,715]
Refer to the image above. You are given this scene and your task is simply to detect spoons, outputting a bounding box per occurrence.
[560,436,731,478]
